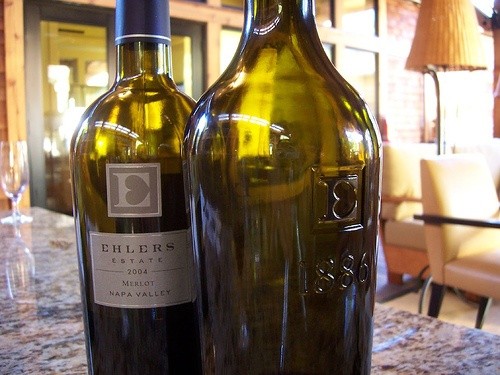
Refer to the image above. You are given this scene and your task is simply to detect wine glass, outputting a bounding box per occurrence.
[4,223,36,303]
[1,140,34,226]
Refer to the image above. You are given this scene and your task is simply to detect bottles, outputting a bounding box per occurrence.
[69,0,217,374]
[184,0,385,375]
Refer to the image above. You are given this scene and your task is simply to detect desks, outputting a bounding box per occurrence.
[0,206,498,374]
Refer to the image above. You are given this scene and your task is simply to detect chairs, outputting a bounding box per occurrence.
[412,154,499,332]
[375,145,442,288]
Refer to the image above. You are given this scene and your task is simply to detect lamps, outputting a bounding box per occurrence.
[404,1,489,159]
